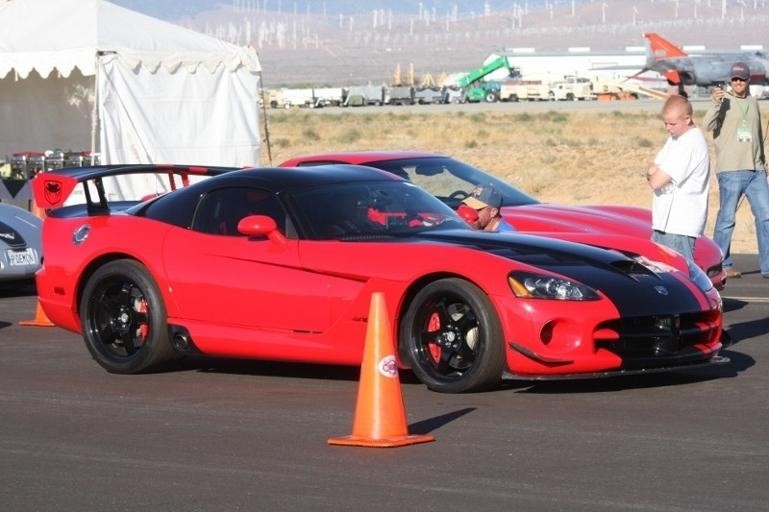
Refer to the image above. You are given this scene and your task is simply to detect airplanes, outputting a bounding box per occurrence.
[642,31,769,87]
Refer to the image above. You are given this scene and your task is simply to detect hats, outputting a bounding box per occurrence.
[730,62,751,80]
[461,184,502,210]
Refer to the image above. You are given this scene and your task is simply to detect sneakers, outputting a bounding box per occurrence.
[726,268,741,278]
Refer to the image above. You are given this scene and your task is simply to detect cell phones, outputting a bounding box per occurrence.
[715,82,723,99]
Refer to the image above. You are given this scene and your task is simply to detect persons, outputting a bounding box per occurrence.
[704,63,768,276]
[460,183,515,232]
[646,95,711,259]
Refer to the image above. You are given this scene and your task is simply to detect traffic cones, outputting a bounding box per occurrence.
[328,292,435,448]
[18,261,57,327]
